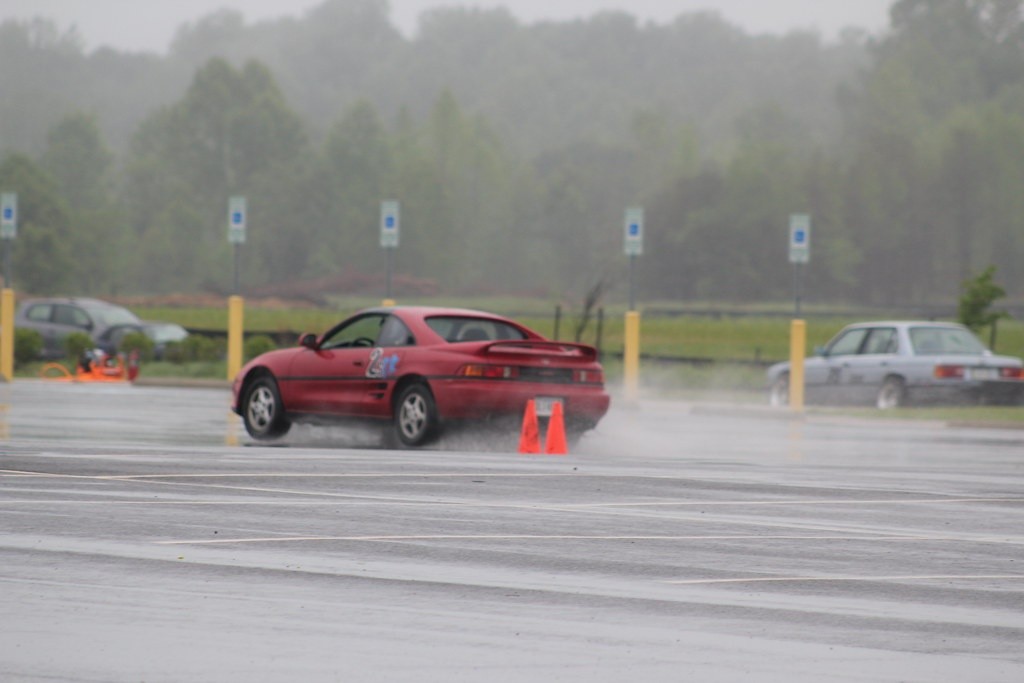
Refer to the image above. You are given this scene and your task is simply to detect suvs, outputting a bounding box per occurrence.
[15,295,189,367]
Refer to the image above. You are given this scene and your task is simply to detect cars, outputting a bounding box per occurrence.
[761,319,1023,414]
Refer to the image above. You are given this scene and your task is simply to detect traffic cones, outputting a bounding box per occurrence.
[542,400,569,454]
[515,398,541,454]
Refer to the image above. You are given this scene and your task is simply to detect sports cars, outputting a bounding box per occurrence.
[231,304,612,450]
